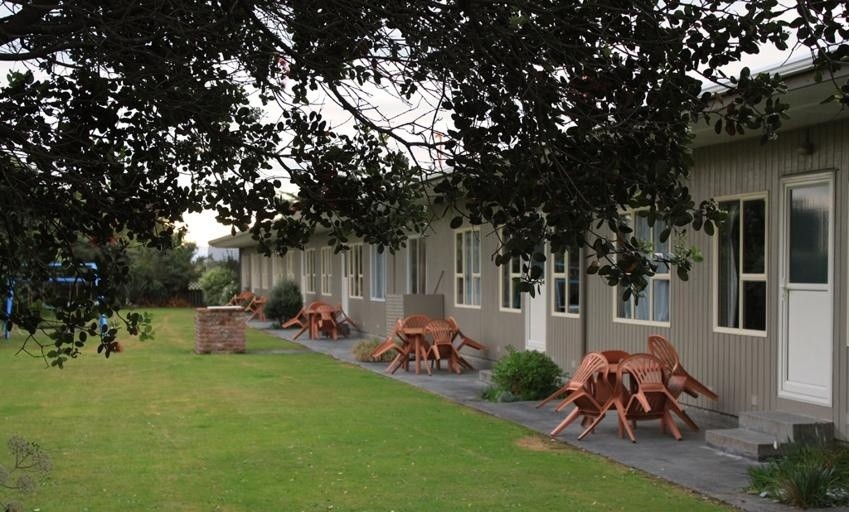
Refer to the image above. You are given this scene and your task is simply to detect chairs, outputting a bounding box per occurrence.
[535,333,722,445]
[369,312,485,378]
[223,290,361,343]
